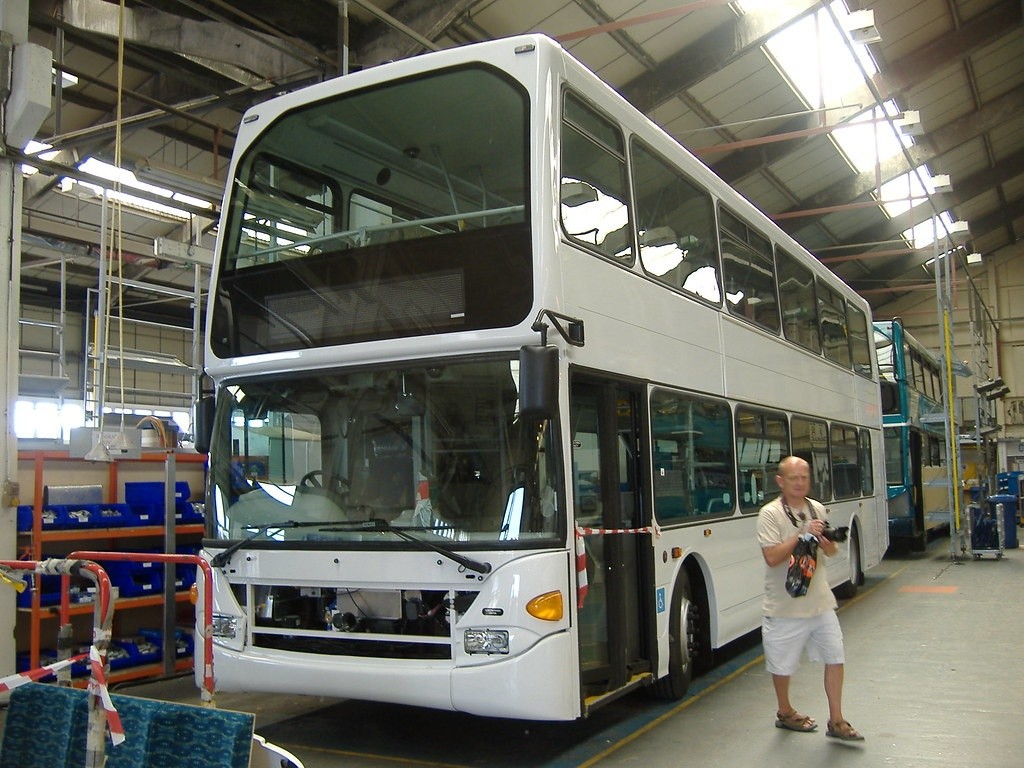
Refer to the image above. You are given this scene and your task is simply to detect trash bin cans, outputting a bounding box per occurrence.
[987,496,1019,550]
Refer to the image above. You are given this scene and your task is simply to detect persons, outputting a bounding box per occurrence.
[756,454,866,741]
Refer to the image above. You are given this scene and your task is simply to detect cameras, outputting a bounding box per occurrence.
[818,521,849,543]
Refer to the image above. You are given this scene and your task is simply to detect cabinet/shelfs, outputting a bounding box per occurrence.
[17,450,208,690]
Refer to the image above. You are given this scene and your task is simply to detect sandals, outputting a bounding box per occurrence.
[825,718,865,742]
[775,709,818,731]
